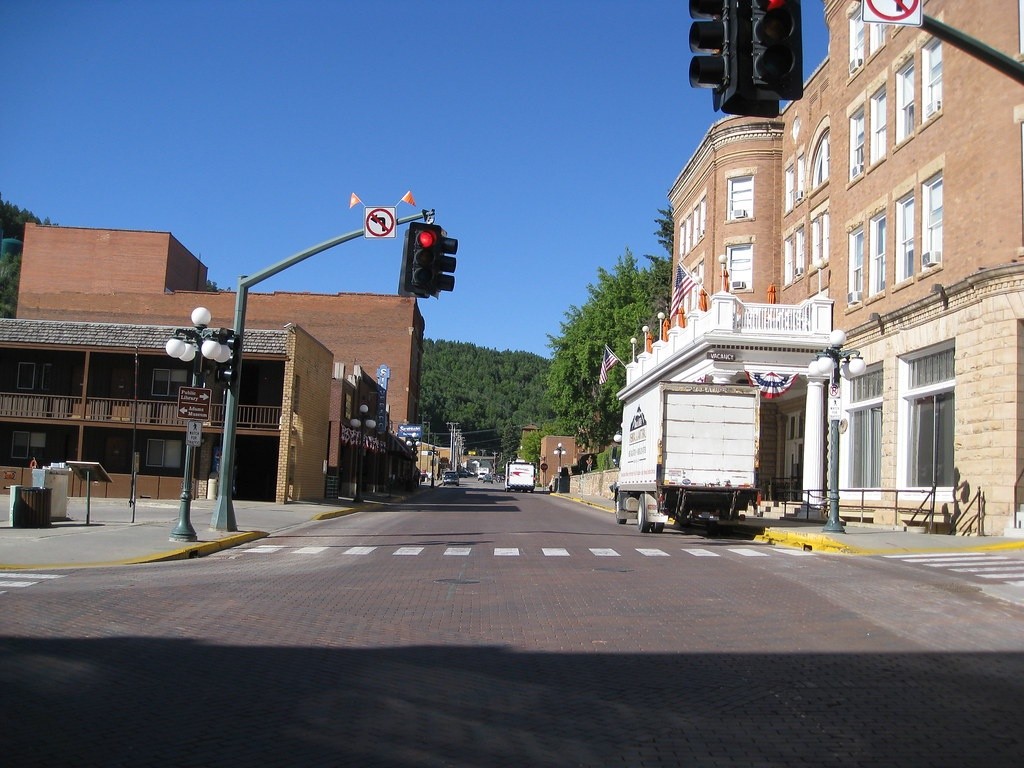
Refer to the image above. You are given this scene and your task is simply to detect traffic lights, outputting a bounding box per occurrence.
[429,225,459,298]
[214,328,240,382]
[405,222,441,298]
[687,0,737,111]
[737,0,803,103]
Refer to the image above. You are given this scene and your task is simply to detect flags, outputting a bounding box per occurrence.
[598,348,618,385]
[670,263,702,320]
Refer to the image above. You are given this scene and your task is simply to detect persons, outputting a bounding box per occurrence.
[586,457,593,472]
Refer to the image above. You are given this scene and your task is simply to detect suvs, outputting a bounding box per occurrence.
[483,474,493,484]
[442,472,459,486]
[478,472,486,481]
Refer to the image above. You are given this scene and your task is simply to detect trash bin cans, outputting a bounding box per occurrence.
[9,485,52,528]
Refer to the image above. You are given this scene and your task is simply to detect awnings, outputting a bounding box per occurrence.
[386,432,418,461]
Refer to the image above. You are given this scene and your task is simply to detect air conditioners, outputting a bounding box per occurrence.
[849,57,863,73]
[927,100,941,117]
[923,250,942,267]
[732,281,745,288]
[852,163,863,178]
[734,209,746,217]
[794,190,804,201]
[847,290,862,306]
[793,267,803,276]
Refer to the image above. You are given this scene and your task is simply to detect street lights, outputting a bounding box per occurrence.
[554,443,566,493]
[349,405,377,503]
[428,446,438,488]
[406,433,420,491]
[807,329,867,536]
[164,308,231,540]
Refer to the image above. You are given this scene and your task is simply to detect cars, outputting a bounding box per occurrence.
[459,471,475,477]
[421,474,428,482]
[495,473,505,482]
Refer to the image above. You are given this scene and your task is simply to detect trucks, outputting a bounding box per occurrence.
[612,379,761,535]
[505,461,536,492]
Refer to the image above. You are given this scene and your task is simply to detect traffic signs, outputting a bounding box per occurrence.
[177,385,212,421]
